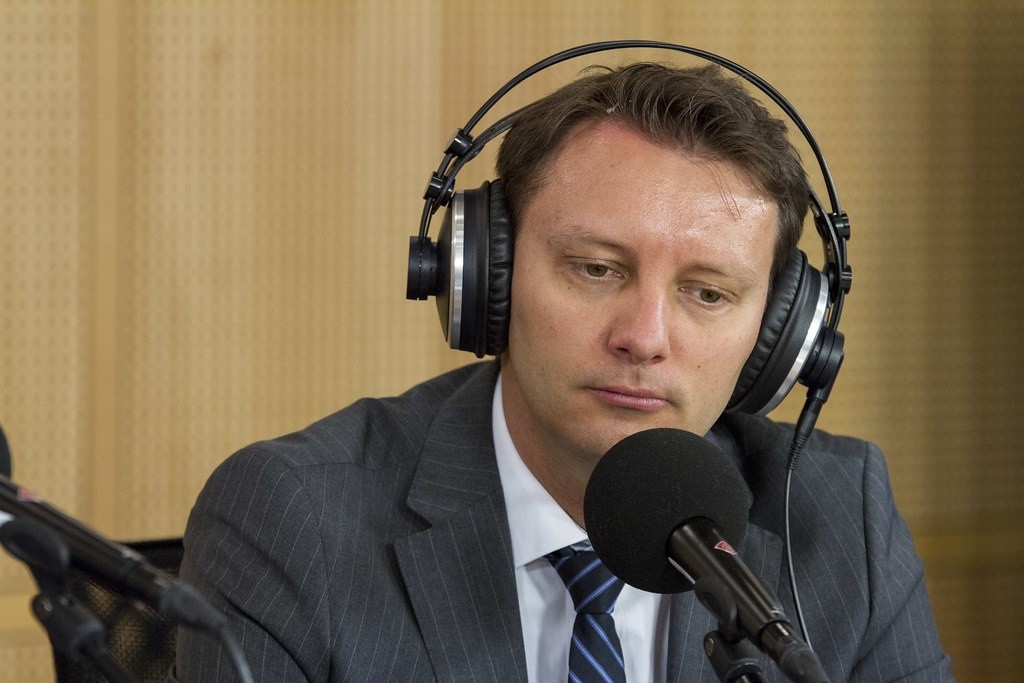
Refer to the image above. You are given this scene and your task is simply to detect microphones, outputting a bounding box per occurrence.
[583,429,831,683]
[0,430,228,634]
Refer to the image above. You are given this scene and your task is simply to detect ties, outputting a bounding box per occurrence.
[544,546,627,683]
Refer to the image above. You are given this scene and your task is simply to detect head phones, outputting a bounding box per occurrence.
[404,39,852,416]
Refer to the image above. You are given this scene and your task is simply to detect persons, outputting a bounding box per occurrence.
[172,55,959,683]
